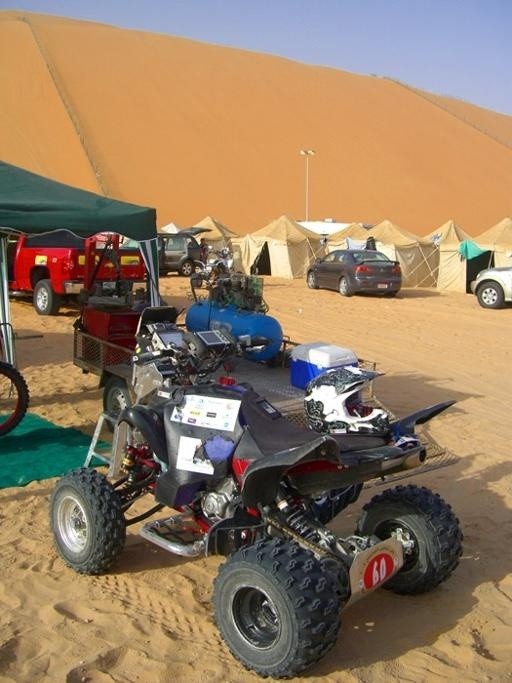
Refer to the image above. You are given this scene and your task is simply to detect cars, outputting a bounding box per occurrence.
[306,248,402,297]
[120,226,213,277]
[470,266,512,309]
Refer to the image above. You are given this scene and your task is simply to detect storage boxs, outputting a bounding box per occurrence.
[289,340,356,393]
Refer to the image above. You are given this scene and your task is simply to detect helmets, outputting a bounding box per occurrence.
[301,364,392,436]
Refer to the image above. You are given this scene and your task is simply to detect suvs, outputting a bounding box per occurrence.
[49,272,465,680]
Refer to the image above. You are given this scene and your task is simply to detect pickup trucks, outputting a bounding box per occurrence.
[6,230,146,315]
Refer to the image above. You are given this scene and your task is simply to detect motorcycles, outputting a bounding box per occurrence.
[189,245,234,288]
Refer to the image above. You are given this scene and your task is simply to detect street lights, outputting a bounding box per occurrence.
[300,149,316,222]
